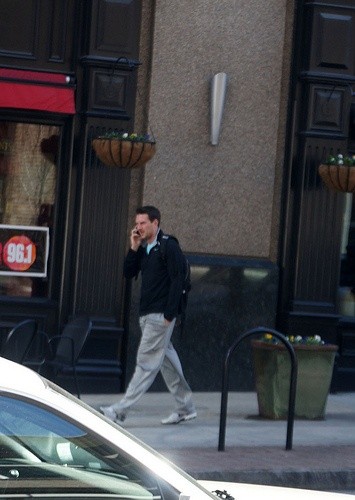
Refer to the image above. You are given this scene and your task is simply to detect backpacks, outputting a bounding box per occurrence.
[160,234,190,296]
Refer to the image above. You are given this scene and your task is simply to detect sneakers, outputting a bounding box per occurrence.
[99,406,126,426]
[161,412,196,424]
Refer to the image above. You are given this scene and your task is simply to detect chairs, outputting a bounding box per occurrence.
[3,315,92,399]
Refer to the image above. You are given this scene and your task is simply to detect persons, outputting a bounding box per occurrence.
[99,205,196,425]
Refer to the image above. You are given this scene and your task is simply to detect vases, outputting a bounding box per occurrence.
[92,139,156,169]
[319,165,355,192]
[253,340,339,420]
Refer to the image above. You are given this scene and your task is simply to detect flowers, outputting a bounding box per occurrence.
[261,333,328,346]
[100,131,156,142]
[323,153,355,166]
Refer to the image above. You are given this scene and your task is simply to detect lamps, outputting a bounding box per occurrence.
[210,72,226,145]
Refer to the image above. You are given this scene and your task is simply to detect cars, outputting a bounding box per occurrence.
[0,357,355,500]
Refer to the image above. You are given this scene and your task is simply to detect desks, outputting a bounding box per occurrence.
[0,321,26,332]
